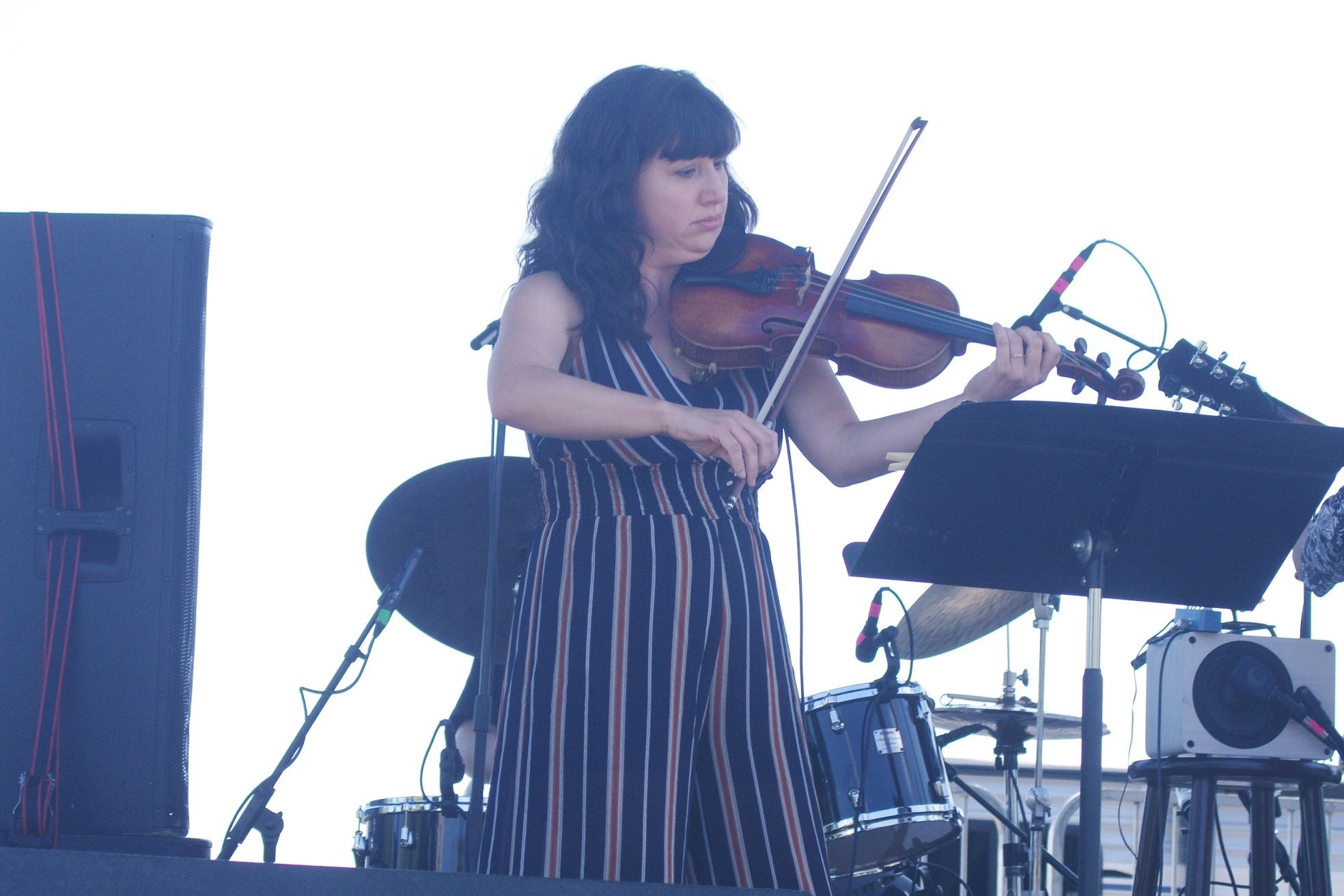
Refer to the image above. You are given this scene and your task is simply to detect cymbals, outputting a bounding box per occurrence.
[365,457,545,653]
[932,705,1111,743]
[890,585,1035,660]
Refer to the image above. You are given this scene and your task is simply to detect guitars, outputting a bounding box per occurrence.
[1157,337,1326,426]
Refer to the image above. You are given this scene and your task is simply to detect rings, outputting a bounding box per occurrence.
[1011,353,1022,359]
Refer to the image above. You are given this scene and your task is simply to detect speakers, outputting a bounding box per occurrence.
[1010,241,1096,332]
[0,211,214,838]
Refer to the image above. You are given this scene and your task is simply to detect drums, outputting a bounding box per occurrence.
[355,796,489,872]
[797,679,964,881]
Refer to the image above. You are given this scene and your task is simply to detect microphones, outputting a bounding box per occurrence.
[1282,696,1338,748]
[373,546,425,638]
[935,724,984,747]
[857,589,885,665]
[442,718,464,784]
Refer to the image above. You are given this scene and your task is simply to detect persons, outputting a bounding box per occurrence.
[1292,485,1344,596]
[471,64,1062,896]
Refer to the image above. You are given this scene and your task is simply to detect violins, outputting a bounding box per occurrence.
[669,222,1146,402]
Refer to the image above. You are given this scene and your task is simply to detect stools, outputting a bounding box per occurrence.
[1127,760,1342,896]
